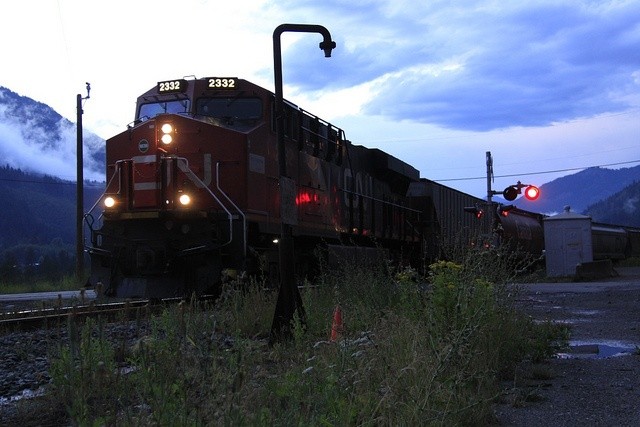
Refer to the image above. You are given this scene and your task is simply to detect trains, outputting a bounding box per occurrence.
[83,75,640,301]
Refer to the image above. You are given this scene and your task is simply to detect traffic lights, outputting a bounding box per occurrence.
[504,185,540,201]
[497,204,516,217]
[463,206,482,219]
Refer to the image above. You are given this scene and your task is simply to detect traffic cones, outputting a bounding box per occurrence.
[332,304,342,340]
